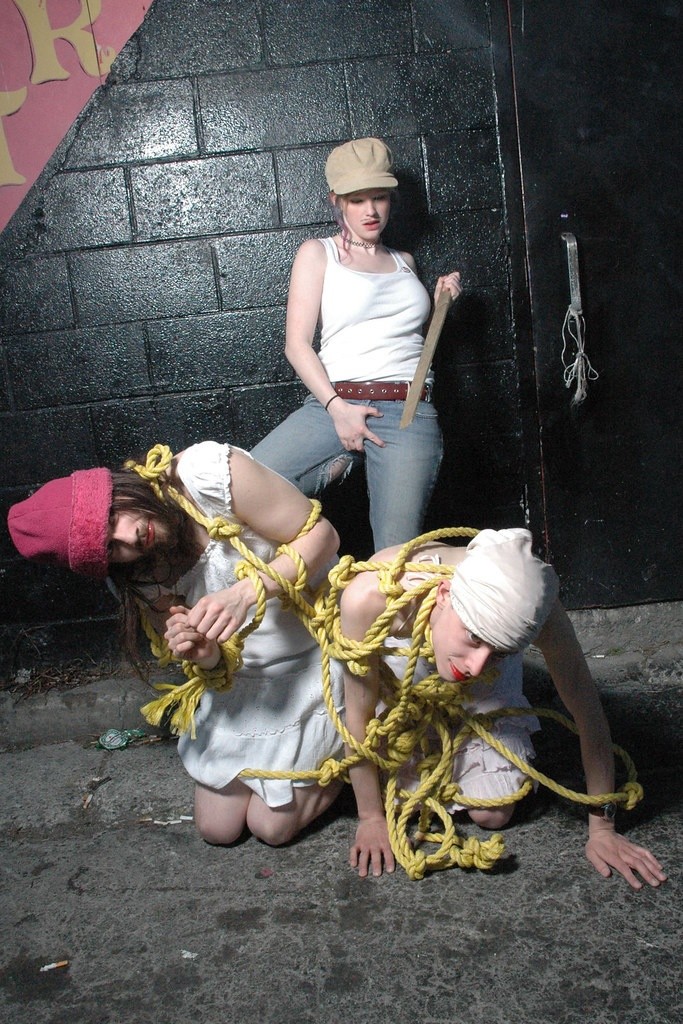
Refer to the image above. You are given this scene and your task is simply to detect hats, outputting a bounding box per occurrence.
[324,138,398,195]
[8,467,112,575]
[450,528,559,653]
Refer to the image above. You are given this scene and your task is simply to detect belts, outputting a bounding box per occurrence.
[330,382,427,400]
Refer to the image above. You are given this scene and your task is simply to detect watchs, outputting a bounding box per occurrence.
[587,802,618,819]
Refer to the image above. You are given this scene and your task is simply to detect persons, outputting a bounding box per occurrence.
[341,527,667,889]
[7,440,350,846]
[246,136,464,559]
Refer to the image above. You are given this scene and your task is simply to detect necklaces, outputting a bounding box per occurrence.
[336,230,382,248]
